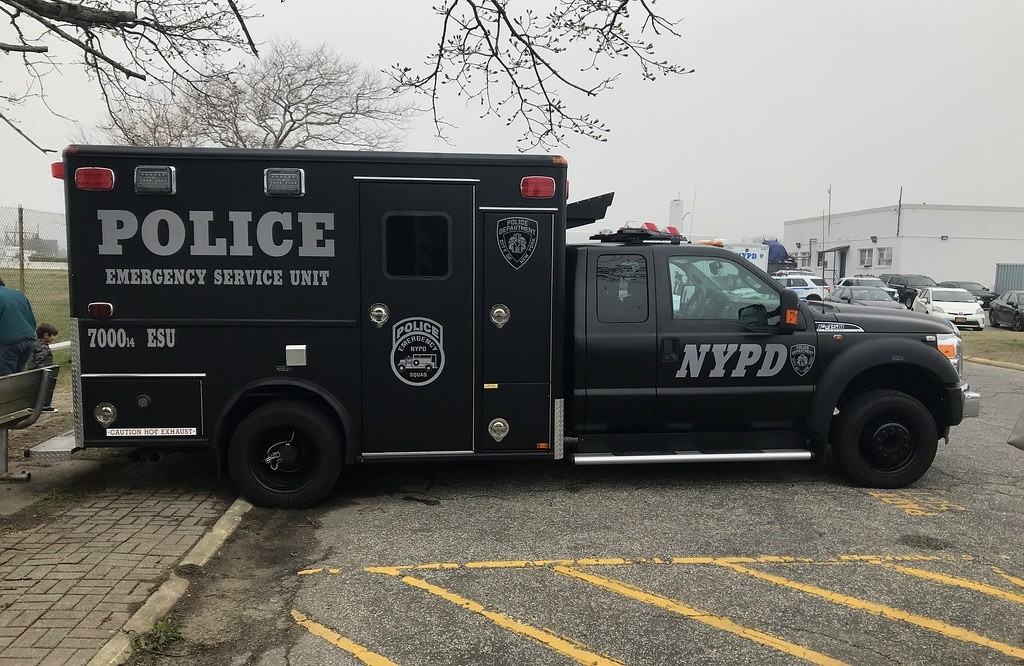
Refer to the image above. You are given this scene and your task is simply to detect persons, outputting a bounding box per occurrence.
[0,278,59,413]
[674,270,685,295]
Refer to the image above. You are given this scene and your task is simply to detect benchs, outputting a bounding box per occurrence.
[0,365,60,481]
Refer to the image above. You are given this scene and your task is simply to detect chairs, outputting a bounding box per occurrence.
[604,279,623,322]
[623,279,646,322]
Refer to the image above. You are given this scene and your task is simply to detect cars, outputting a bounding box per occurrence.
[824,286,907,311]
[939,281,994,308]
[911,288,985,331]
[837,274,899,302]
[771,275,832,301]
[989,290,1023,331]
[878,274,940,308]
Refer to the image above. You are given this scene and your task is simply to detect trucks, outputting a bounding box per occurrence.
[52,146,981,509]
[994,263,1023,295]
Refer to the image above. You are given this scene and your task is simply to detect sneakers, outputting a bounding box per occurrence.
[27,407,34,412]
[41,407,58,413]
